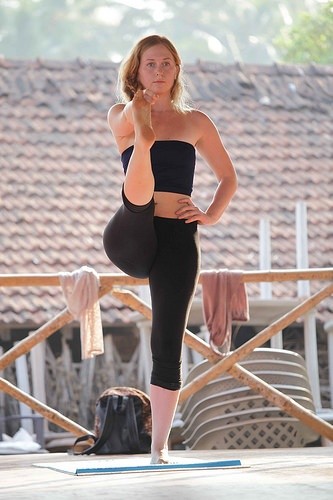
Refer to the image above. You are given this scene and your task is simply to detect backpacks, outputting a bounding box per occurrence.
[71,387,152,455]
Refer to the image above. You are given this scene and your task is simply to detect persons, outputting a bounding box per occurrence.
[102,35,238,467]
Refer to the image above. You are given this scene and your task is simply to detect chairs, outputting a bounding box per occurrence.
[178,346,322,453]
